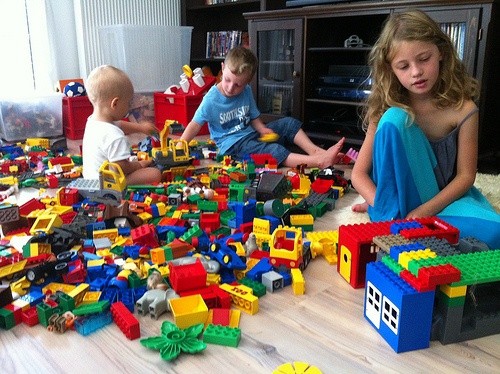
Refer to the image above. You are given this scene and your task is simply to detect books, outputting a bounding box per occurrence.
[437,22,465,65]
[256,30,294,81]
[256,82,293,114]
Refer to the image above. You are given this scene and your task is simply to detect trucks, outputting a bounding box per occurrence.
[65,159,128,203]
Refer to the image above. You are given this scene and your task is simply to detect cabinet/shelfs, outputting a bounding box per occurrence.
[243,0,500,163]
[180,0,286,76]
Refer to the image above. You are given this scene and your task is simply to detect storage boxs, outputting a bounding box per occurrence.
[97,23,194,93]
[0,79,94,155]
[153,75,215,137]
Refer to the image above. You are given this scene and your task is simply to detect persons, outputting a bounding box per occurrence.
[209,32,232,57]
[351,8,500,250]
[176,45,345,172]
[81,64,162,185]
